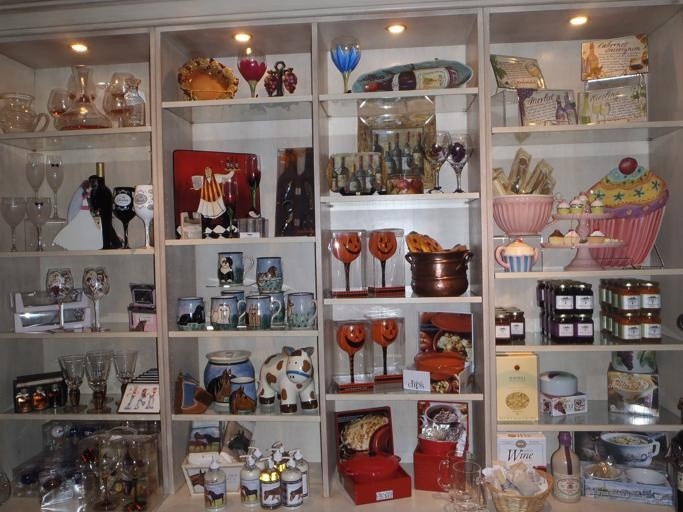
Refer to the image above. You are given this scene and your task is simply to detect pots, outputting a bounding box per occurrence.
[402,246,474,298]
[340,449,401,483]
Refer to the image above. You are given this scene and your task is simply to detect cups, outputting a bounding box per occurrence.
[492,192,668,273]
[435,450,489,512]
[46,88,72,118]
[323,228,408,392]
[176,249,316,330]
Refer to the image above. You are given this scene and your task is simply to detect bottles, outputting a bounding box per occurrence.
[366,68,457,91]
[228,375,263,414]
[586,41,599,75]
[673,448,683,512]
[332,155,382,193]
[373,130,426,177]
[277,148,315,235]
[203,349,257,406]
[54,64,148,130]
[89,160,113,250]
[549,431,581,504]
[554,90,592,125]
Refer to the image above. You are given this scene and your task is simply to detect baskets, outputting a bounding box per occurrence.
[487,467,554,512]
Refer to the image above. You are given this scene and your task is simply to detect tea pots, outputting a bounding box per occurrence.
[0,92,51,134]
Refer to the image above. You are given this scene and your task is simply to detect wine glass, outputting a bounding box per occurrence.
[0,151,66,252]
[56,347,140,413]
[45,267,74,335]
[329,34,362,95]
[592,102,611,125]
[442,134,475,193]
[422,129,453,194]
[235,47,266,98]
[80,266,114,334]
[244,154,262,218]
[72,427,154,512]
[525,63,544,88]
[222,177,240,237]
[111,183,154,249]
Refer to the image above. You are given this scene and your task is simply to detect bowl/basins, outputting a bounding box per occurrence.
[612,377,652,401]
[416,432,459,456]
[423,405,465,427]
[539,371,578,397]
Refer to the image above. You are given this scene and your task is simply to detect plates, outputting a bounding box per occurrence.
[584,431,668,488]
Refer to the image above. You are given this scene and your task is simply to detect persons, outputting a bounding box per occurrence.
[145,386,158,409]
[190,164,239,239]
[133,387,149,409]
[53,178,103,252]
[122,384,141,409]
[88,175,124,249]
